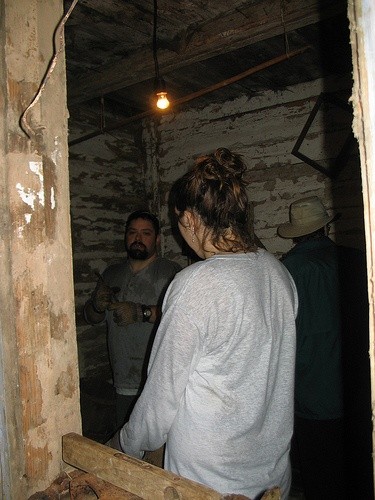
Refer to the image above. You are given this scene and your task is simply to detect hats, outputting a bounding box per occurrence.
[278,196,342,240]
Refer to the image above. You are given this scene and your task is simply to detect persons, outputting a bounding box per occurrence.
[82,211,182,467]
[105,146,299,499]
[272,196,370,499]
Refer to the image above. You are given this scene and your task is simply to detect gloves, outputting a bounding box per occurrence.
[93,283,119,311]
[107,302,143,327]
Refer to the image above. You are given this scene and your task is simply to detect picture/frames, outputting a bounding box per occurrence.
[290,94,353,180]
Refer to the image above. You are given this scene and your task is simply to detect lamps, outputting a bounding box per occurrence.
[153,0,171,109]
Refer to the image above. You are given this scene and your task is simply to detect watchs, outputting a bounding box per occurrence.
[141,302,153,323]
